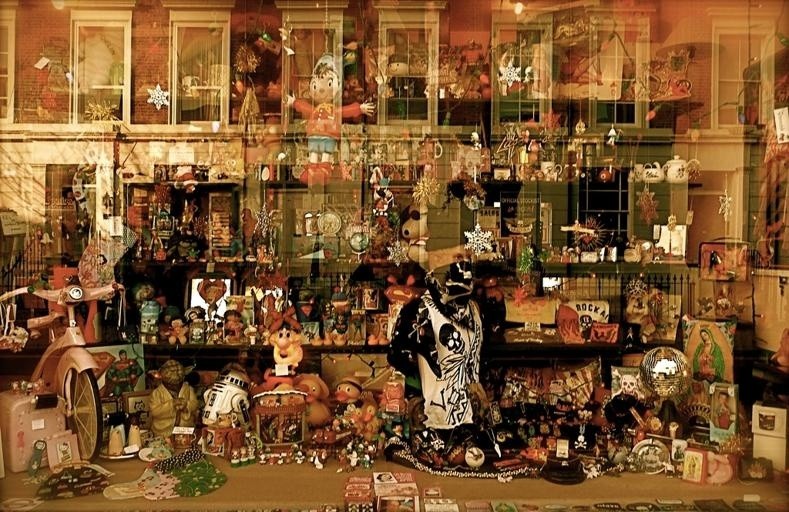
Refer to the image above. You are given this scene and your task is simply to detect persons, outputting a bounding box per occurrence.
[148,360,200,439]
[286,52,375,187]
